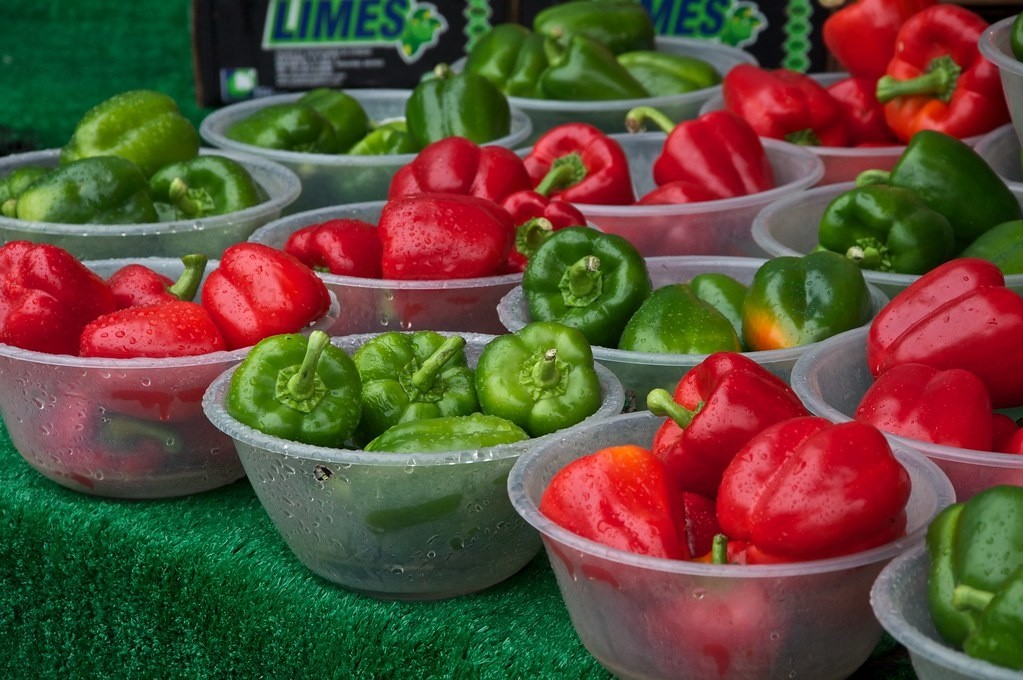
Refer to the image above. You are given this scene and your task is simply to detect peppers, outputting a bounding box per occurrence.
[0,0,1023,680]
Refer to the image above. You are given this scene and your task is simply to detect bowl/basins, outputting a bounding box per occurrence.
[0,147,302,259]
[0,260,339,499]
[501,133,824,257]
[701,10,1023,208]
[507,410,957,680]
[450,37,758,134]
[200,88,533,216]
[869,541,1023,680]
[790,321,1023,502]
[247,198,605,337]
[751,181,1023,305]
[203,330,626,602]
[497,256,891,415]
